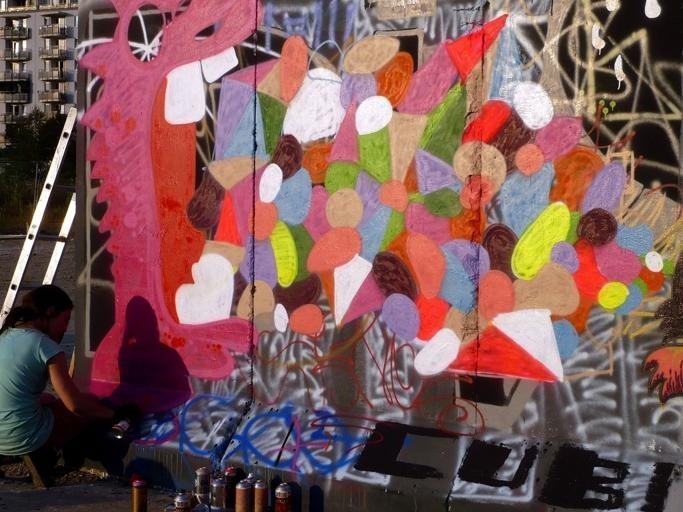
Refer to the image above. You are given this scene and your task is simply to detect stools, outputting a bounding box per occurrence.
[0,454,46,488]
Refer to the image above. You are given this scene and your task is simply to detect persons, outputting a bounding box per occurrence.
[0,283,143,482]
